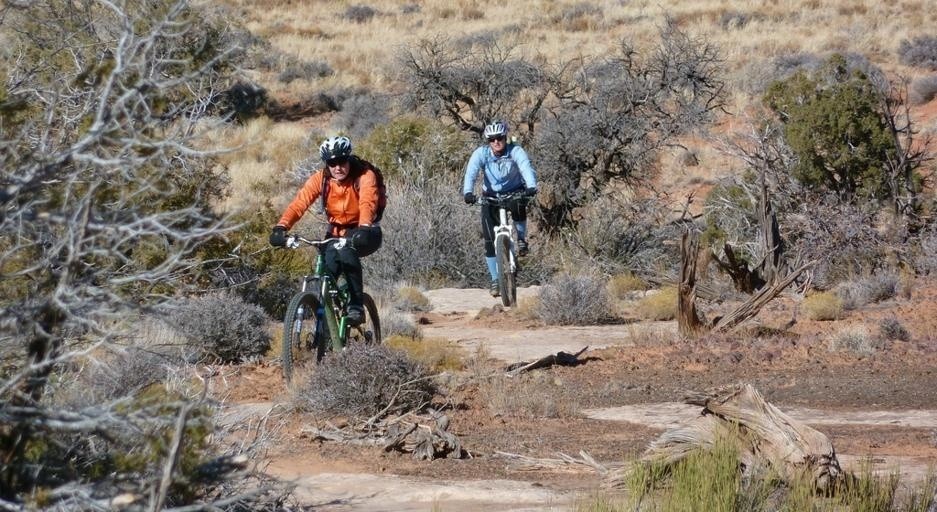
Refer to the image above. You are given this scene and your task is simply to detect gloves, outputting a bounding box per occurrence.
[268,225,287,247]
[351,225,371,250]
[463,192,476,204]
[525,187,537,197]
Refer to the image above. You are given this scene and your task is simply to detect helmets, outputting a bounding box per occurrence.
[483,123,507,139]
[318,135,353,161]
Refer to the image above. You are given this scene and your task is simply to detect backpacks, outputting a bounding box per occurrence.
[319,154,388,223]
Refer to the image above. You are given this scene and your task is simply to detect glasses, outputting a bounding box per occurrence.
[488,136,504,142]
[325,158,348,167]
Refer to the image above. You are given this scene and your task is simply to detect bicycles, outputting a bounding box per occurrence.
[274,233,382,385]
[466,189,538,306]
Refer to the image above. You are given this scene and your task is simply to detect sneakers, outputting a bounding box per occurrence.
[490,278,499,297]
[517,238,529,256]
[344,304,366,325]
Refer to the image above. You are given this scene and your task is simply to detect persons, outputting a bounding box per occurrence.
[268,135,382,351]
[461,120,538,298]
